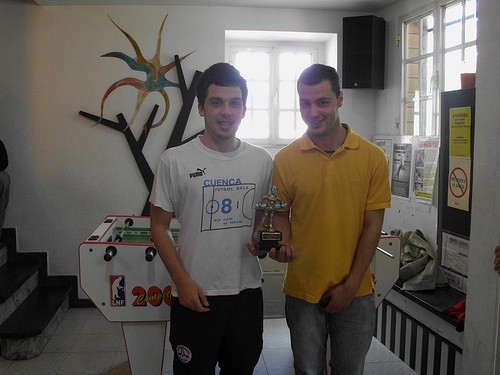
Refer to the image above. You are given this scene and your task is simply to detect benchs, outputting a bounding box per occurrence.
[370,276,466,375]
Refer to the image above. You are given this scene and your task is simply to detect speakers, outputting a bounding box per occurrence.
[342,16,385,91]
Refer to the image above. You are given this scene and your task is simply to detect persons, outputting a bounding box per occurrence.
[269,64,392,375]
[394,156,407,180]
[148,63,273,375]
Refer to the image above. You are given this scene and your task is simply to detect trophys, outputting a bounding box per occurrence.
[255,185,288,251]
